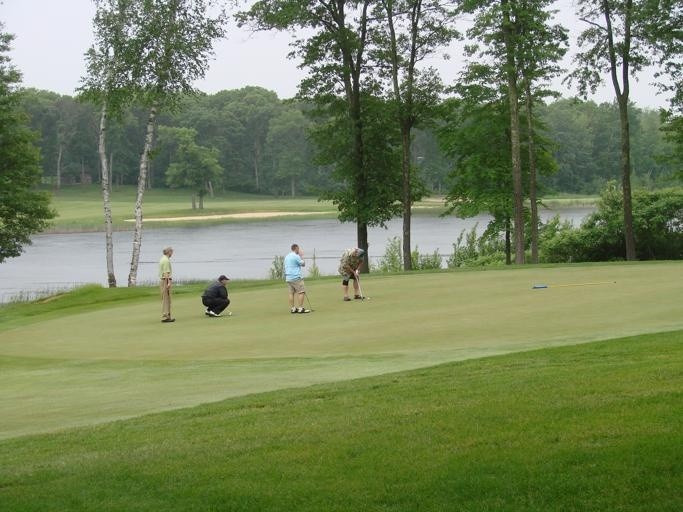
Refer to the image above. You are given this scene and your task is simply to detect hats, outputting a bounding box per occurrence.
[218,275,229,282]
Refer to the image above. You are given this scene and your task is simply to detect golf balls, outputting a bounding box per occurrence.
[367,297,370,300]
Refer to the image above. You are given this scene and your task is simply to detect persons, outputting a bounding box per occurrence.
[201,275,230,317]
[283,244,310,313]
[158,247,175,322]
[337,247,366,301]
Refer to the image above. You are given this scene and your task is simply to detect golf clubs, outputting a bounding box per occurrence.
[355,275,365,304]
[304,293,315,312]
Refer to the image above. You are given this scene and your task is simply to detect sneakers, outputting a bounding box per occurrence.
[161,318,176,322]
[291,306,310,313]
[205,309,220,317]
[354,295,365,299]
[344,296,350,301]
